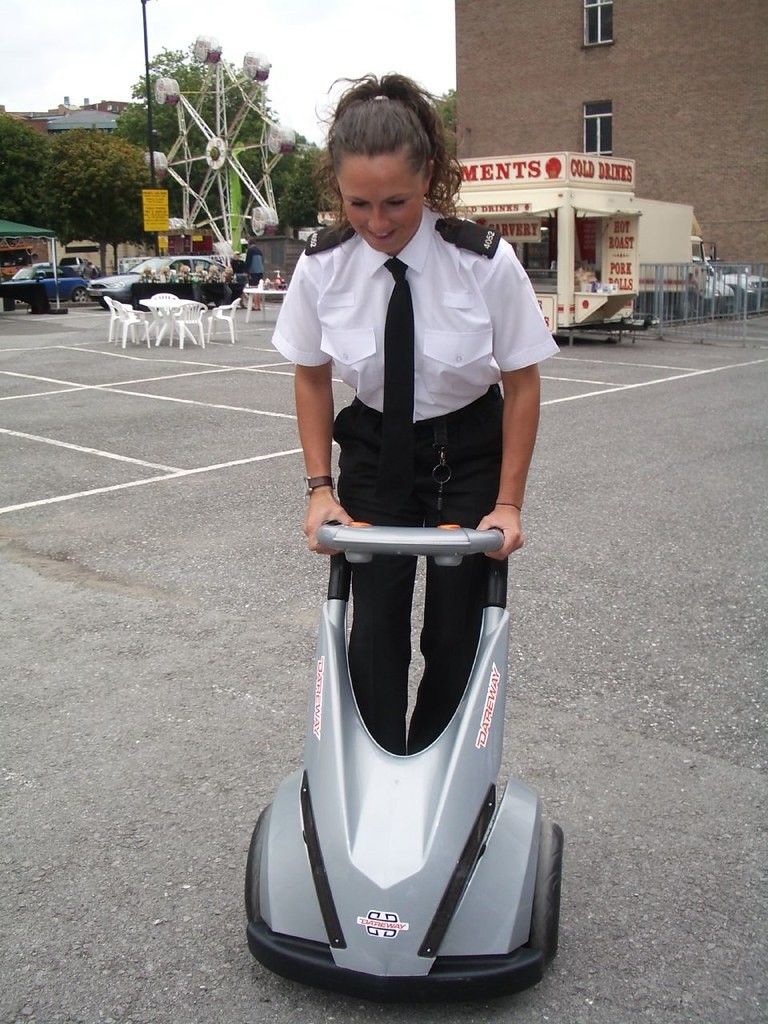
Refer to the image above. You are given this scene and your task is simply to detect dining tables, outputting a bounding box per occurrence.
[243,287,287,323]
[139,299,198,347]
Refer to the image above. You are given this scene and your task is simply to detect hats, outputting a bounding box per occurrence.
[234,251,240,254]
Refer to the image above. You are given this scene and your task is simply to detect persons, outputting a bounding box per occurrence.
[231,251,247,309]
[271,74,561,756]
[245,239,264,311]
[86,262,101,279]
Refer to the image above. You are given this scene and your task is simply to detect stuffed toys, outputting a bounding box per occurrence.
[137,264,233,283]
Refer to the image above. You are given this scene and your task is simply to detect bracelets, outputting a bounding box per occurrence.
[496,503,521,511]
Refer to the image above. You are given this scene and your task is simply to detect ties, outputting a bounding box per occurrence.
[372,258,426,514]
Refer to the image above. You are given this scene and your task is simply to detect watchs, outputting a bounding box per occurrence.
[302,475,336,496]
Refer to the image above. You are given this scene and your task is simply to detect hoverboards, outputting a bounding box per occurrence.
[240,521,563,1005]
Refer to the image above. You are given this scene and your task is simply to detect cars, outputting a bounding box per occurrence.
[691,236,767,313]
[59,256,95,280]
[0,263,89,303]
[87,256,227,312]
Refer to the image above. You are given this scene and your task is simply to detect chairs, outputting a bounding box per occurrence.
[206,298,241,344]
[103,292,208,350]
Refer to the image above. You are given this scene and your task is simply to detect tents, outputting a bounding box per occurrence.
[0,218,60,310]
